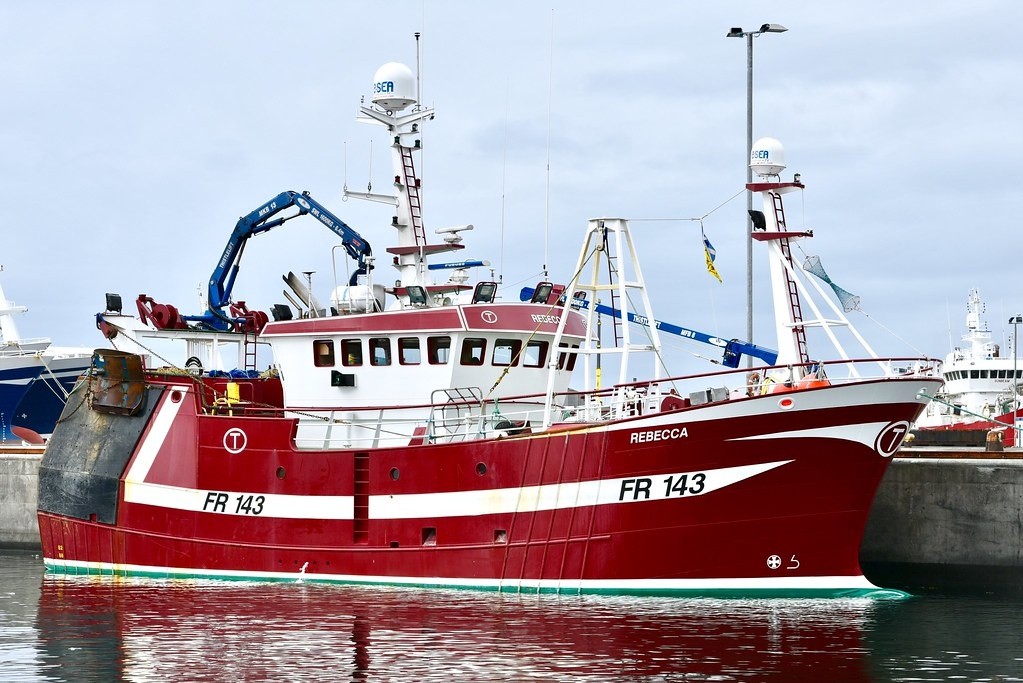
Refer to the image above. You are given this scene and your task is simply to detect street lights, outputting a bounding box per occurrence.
[725,24,792,397]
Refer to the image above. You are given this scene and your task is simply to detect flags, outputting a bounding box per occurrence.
[703,234,722,284]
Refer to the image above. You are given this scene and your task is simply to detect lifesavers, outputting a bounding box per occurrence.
[748,374,765,398]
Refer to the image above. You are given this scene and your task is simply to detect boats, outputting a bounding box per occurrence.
[0,266,104,458]
[35,36,948,601]
[896,291,1023,458]
